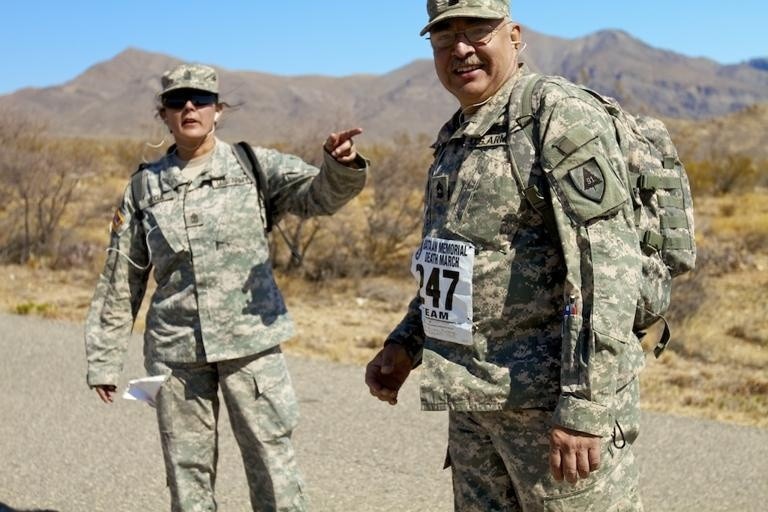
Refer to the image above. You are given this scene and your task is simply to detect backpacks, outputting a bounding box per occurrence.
[507,70,696,331]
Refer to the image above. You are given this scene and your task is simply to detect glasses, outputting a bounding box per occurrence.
[426,21,510,49]
[163,89,218,109]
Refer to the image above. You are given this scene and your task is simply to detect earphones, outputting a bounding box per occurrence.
[164,117,168,126]
[214,111,219,121]
[511,28,520,49]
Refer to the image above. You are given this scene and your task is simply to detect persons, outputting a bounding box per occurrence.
[360,0,651,511]
[82,60,371,510]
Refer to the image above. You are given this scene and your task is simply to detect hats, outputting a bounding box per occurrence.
[420,0,510,36]
[157,63,218,97]
